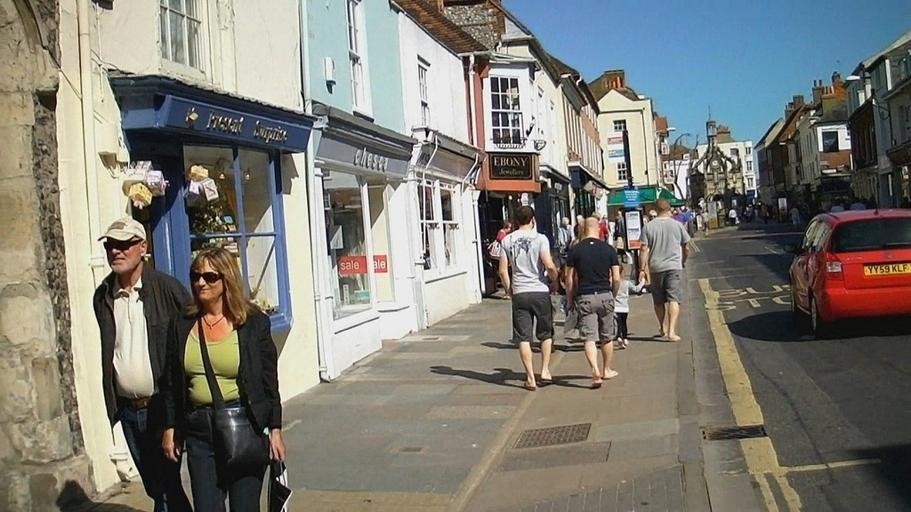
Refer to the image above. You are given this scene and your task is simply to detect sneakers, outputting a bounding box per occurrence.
[624,339,628,346]
[603,371,617,378]
[592,378,602,386]
[617,337,625,349]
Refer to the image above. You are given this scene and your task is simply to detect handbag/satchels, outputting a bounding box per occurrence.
[485,240,502,260]
[215,405,269,466]
[267,458,293,512]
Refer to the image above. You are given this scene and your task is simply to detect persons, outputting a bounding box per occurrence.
[162,247,285,512]
[554,209,646,389]
[497,206,559,390]
[726,198,878,227]
[638,200,709,341]
[93,219,193,512]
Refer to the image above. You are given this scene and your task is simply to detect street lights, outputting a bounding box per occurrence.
[845,75,871,82]
[653,126,676,188]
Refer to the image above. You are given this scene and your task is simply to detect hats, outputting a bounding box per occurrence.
[98,217,147,241]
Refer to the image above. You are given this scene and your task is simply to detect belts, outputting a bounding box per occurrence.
[119,397,150,409]
[581,290,608,294]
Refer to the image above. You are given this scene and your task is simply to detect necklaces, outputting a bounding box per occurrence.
[202,315,226,330]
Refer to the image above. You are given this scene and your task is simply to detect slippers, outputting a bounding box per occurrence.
[540,377,556,384]
[668,336,680,341]
[524,383,536,390]
[653,334,668,341]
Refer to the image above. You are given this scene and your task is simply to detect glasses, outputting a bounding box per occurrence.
[103,238,142,249]
[189,271,221,282]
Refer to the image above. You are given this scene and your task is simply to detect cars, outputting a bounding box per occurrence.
[784,209,910,339]
